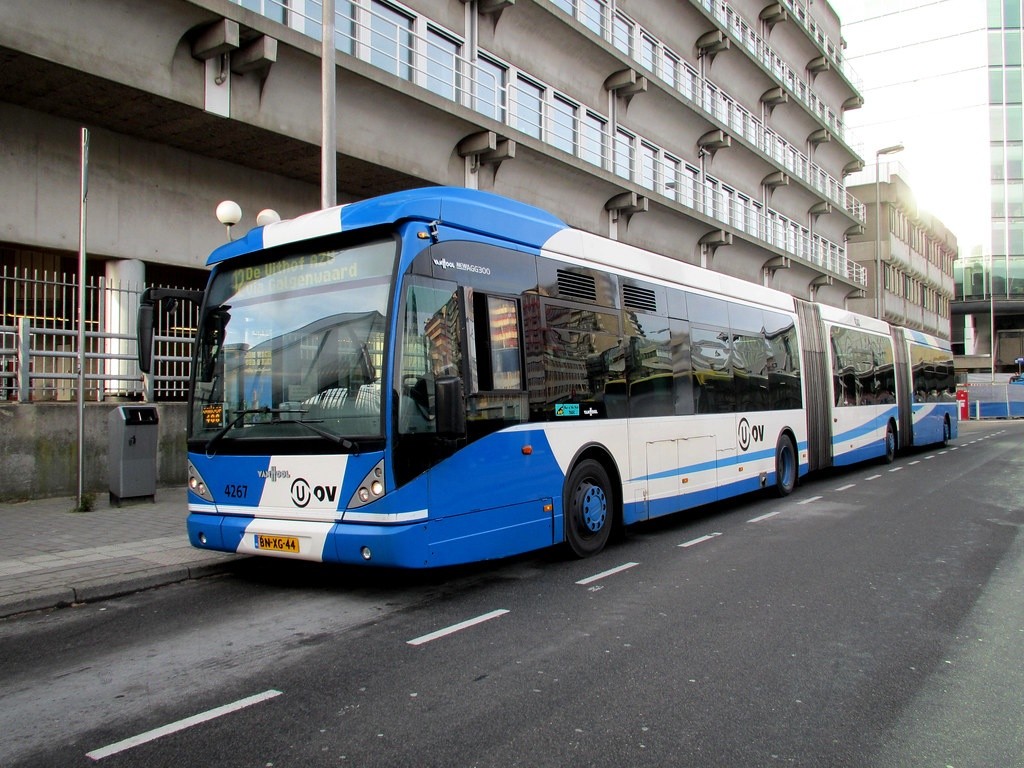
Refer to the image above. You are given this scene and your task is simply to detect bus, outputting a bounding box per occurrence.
[183,185,960,568]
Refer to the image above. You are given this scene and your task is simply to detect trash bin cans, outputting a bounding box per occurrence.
[107,406,160,509]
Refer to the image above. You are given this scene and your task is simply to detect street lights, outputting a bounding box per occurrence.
[875,144,905,320]
